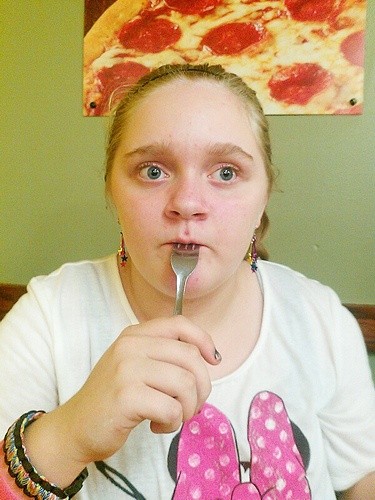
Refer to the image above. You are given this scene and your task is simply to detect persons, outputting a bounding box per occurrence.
[1,61,375,499]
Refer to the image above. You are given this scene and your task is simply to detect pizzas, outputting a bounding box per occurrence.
[83,0,366,117]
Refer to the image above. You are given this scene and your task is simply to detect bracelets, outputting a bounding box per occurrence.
[3,407,91,500]
[0,472,21,499]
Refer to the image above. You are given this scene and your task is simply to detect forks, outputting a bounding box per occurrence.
[170,242,200,315]
